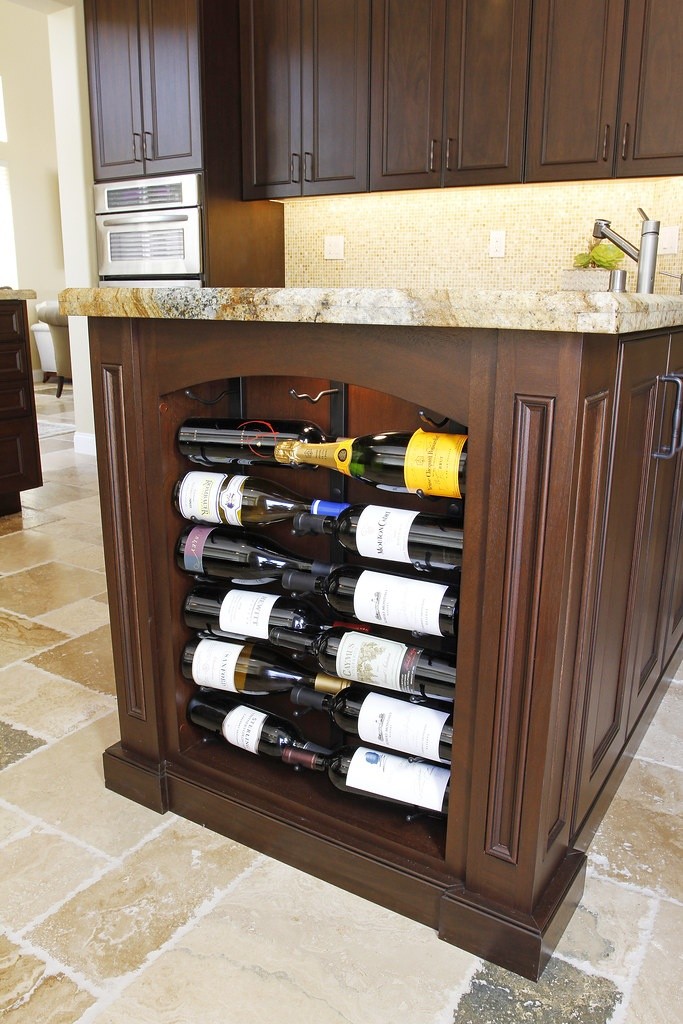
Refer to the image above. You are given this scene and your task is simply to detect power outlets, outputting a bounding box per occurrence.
[323,235,344,259]
[489,230,506,258]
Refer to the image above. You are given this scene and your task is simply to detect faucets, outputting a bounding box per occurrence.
[592,208,662,294]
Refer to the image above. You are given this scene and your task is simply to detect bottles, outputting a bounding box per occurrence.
[176,418,467,816]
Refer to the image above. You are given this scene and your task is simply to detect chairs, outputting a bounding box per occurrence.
[30,323,58,383]
[35,300,73,397]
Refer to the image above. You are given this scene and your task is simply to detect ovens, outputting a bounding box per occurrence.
[93,172,286,289]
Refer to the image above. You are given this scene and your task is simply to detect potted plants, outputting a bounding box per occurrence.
[560,241,624,292]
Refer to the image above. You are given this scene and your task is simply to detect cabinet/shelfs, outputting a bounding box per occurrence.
[0,299,41,517]
[77,0,683,986]
